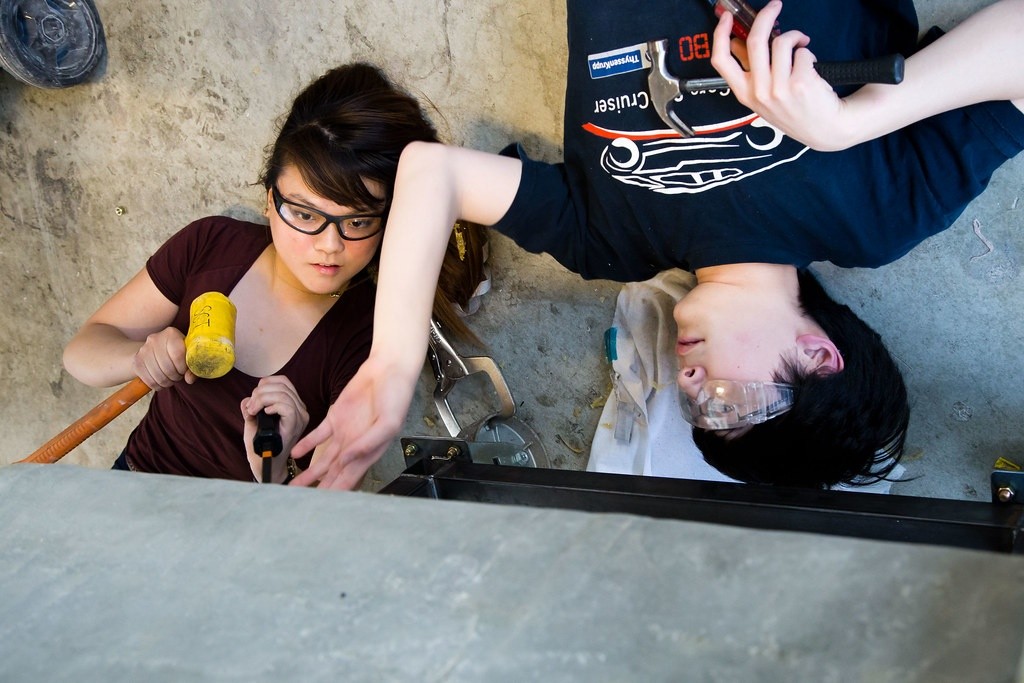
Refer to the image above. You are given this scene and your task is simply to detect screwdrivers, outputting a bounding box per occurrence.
[253,406,284,483]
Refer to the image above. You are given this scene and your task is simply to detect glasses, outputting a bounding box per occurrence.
[271,181,388,241]
[676,379,795,430]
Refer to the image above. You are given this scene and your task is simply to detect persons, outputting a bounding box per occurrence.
[64,64,479,485]
[287,0,1024,491]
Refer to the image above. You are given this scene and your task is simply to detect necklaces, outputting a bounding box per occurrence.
[288,283,342,298]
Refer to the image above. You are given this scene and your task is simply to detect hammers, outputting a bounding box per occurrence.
[11,292,240,466]
